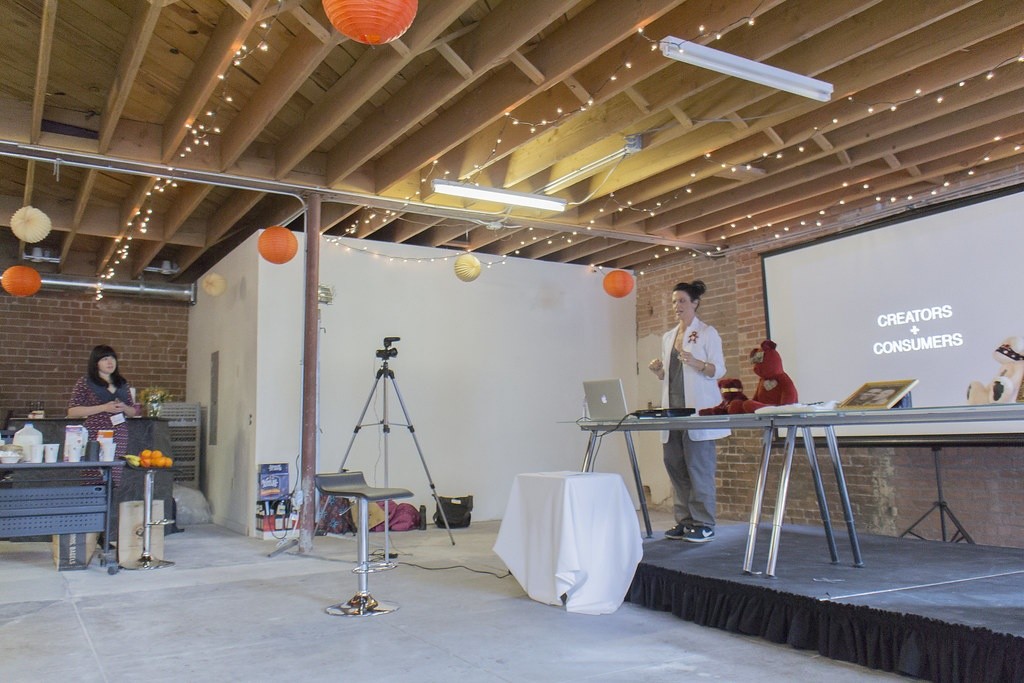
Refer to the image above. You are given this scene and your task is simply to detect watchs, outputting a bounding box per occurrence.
[698,360,707,372]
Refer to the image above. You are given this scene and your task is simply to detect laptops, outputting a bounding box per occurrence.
[583,378,638,420]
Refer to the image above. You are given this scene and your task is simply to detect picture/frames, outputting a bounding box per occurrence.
[837,378,919,409]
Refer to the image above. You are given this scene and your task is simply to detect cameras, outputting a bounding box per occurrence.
[375,337,400,360]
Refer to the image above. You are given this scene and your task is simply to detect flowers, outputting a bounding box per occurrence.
[138,384,173,404]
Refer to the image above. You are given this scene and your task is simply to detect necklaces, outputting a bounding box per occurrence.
[100,377,113,384]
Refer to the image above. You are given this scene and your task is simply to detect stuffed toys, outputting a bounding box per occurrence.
[698,378,748,416]
[728,340,798,414]
[966,337,1024,405]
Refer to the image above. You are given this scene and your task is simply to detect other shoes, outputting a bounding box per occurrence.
[97,535,115,550]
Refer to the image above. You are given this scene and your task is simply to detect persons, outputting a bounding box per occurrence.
[68,344,140,549]
[851,387,898,406]
[648,280,727,542]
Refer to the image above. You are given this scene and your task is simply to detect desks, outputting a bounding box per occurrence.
[579,406,1024,575]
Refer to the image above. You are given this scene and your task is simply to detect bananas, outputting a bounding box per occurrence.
[119,454,141,467]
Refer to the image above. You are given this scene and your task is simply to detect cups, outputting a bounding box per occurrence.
[30,444,43,464]
[86,441,99,462]
[102,443,117,462]
[45,444,60,463]
[68,444,82,463]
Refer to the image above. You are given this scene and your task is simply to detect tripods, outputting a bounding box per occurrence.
[311,360,455,546]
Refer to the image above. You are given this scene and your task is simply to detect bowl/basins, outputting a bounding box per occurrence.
[0,456,22,463]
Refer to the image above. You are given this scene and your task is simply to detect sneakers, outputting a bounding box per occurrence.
[664,523,715,542]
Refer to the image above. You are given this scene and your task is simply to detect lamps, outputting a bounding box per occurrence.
[659,36,834,103]
[431,178,568,212]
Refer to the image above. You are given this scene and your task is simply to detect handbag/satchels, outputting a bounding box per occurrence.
[319,496,357,536]
[432,494,474,529]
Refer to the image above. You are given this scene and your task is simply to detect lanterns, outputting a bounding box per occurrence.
[603,270,635,298]
[321,0,418,44]
[455,254,481,282]
[1,266,41,297]
[258,226,298,264]
[10,206,51,243]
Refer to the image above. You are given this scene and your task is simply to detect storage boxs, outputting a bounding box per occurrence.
[257,463,289,501]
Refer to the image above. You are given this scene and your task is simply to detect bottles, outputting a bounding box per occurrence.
[12,423,42,462]
[420,504,426,530]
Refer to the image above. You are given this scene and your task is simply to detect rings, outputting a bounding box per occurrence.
[685,359,688,362]
[686,356,687,359]
[686,352,688,356]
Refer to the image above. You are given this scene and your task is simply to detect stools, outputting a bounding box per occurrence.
[315,472,414,618]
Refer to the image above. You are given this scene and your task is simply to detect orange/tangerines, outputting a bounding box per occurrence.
[139,449,173,467]
